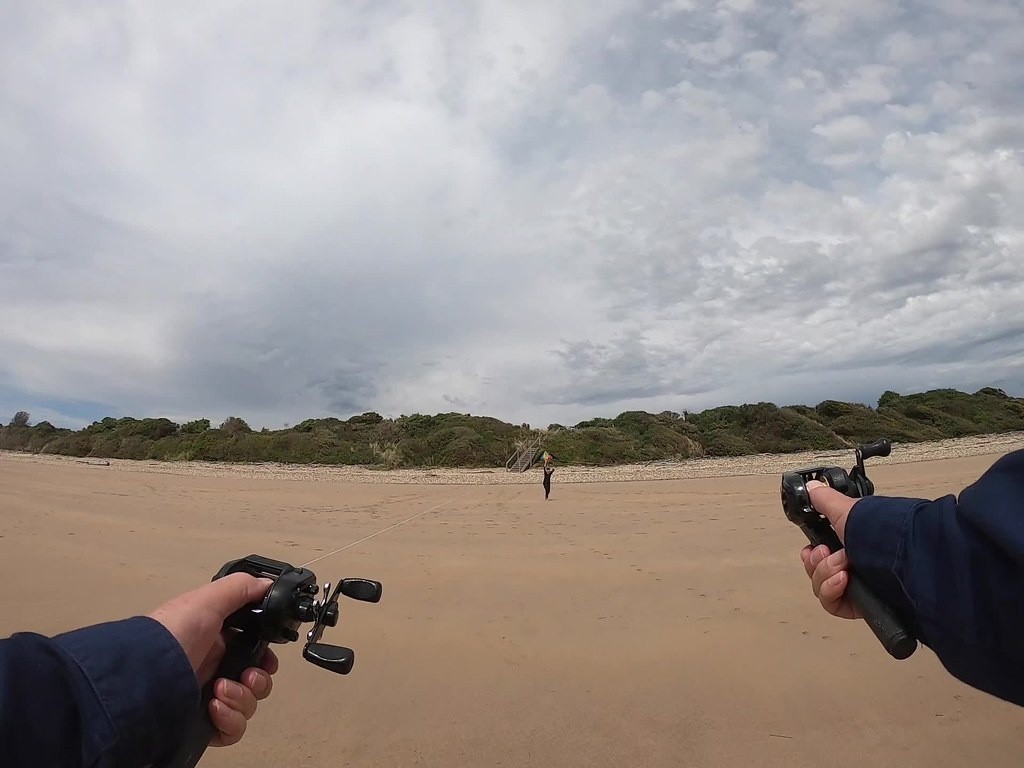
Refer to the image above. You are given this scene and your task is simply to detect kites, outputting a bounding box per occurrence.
[540,451,553,462]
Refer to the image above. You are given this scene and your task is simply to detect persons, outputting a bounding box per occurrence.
[1,446,1024,767]
[542,460,555,500]
